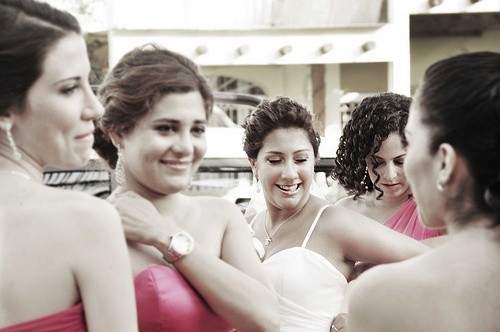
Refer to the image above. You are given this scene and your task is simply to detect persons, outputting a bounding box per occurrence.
[92,42,281,332]
[240,96,431,332]
[344,52,500,332]
[329,91,448,240]
[0,0,138,332]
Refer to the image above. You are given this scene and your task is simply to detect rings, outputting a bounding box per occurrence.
[332,325,339,331]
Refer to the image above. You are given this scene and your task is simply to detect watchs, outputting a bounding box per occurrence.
[161,230,195,263]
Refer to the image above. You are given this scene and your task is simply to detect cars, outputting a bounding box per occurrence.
[205,105,249,159]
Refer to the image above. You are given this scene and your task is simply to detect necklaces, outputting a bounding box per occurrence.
[261,208,298,246]
